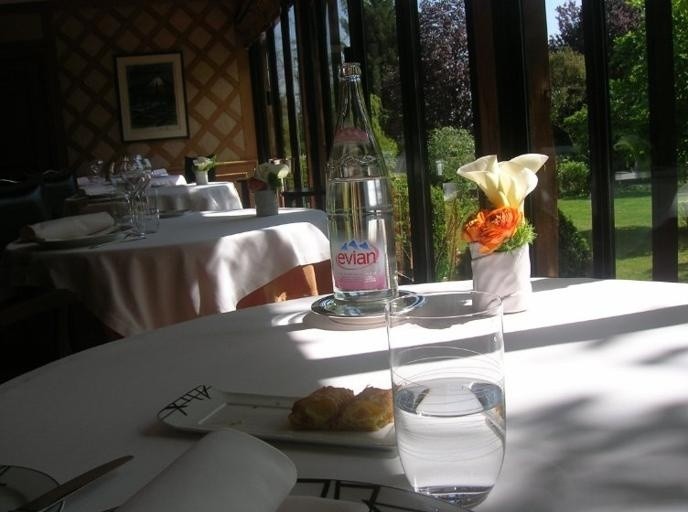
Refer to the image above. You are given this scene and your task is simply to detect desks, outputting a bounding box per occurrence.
[0,168,688,512]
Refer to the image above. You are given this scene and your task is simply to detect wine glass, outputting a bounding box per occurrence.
[109,157,153,241]
[90,158,103,183]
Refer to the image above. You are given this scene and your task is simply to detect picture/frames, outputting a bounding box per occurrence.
[113,51,189,144]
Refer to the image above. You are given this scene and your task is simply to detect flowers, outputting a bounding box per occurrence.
[456,153,551,255]
[247,158,291,192]
[191,152,216,172]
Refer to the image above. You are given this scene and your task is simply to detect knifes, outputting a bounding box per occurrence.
[10,455,132,512]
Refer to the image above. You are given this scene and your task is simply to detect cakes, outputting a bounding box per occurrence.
[290,386,354,430]
[338,388,393,431]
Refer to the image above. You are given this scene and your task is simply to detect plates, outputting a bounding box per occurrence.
[311,290,426,329]
[45,234,99,247]
[158,383,397,453]
[0,462,65,511]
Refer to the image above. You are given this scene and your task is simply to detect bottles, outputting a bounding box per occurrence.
[324,62,397,313]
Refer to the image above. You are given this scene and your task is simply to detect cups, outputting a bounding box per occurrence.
[384,291,507,506]
